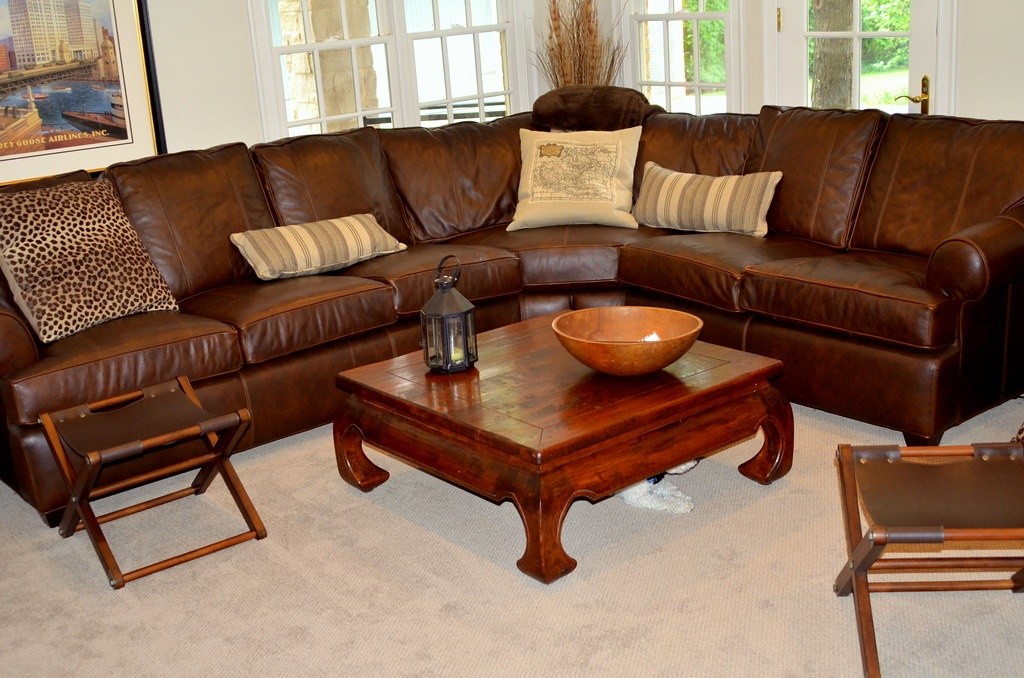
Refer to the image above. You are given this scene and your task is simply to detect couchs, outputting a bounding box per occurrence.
[0,85,1024,529]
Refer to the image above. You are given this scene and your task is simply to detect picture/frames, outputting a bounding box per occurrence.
[0,0,168,188]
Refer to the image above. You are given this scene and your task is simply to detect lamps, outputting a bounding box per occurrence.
[420,254,479,376]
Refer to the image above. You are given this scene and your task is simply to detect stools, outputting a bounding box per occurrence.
[37,375,269,591]
[831,442,1024,678]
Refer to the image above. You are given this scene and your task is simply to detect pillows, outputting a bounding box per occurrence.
[744,103,891,251]
[104,142,277,305]
[0,170,92,299]
[506,125,643,232]
[376,111,533,245]
[229,213,408,281]
[0,177,181,343]
[631,160,783,239]
[848,112,1024,260]
[632,110,759,206]
[248,126,416,246]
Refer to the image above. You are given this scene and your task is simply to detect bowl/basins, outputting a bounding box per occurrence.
[550,305,704,376]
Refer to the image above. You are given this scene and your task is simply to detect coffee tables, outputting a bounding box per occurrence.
[332,308,796,584]
